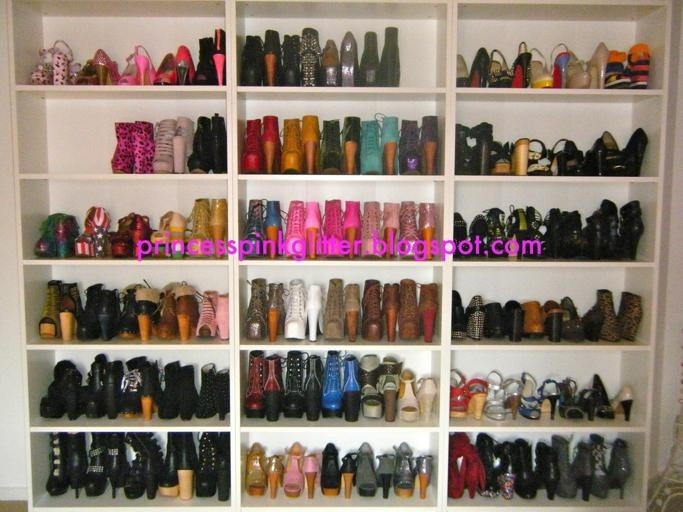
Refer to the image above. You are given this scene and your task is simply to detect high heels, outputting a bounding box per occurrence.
[244,200,436,258]
[245,351,436,421]
[450,369,634,421]
[39,279,229,340]
[242,28,399,87]
[31,26,225,86]
[240,115,437,173]
[454,200,643,260]
[448,432,629,500]
[112,114,227,174]
[456,42,651,88]
[246,442,432,499]
[35,199,227,259]
[452,290,643,341]
[454,122,648,176]
[41,354,230,421]
[46,432,230,501]
[245,279,437,342]
